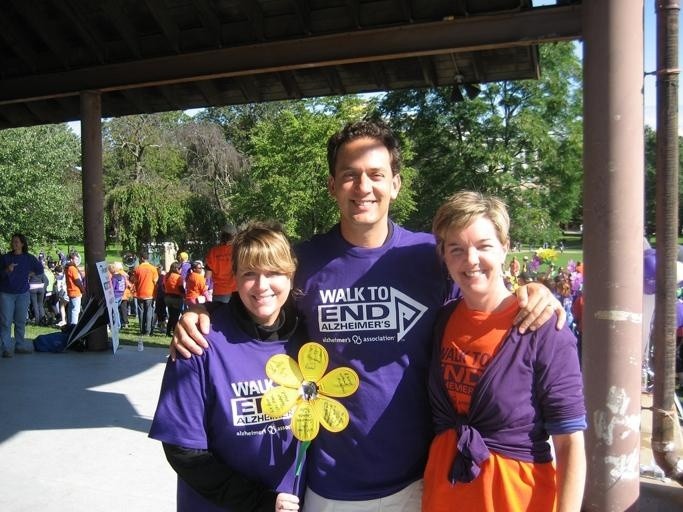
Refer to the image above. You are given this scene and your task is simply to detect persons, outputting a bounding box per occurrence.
[497,234,683,398]
[169,121,568,512]
[0,234,44,358]
[194,260,206,278]
[132,252,159,336]
[113,261,131,329]
[48,262,61,327]
[46,256,54,268]
[27,261,49,324]
[205,231,239,303]
[108,264,126,332]
[162,262,187,337]
[177,250,192,281]
[421,190,587,512]
[185,263,211,305]
[56,250,66,266]
[148,221,316,512]
[64,254,85,327]
[53,266,69,327]
[39,251,44,262]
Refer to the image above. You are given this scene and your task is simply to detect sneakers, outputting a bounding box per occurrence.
[16,348,33,353]
[4,352,13,358]
